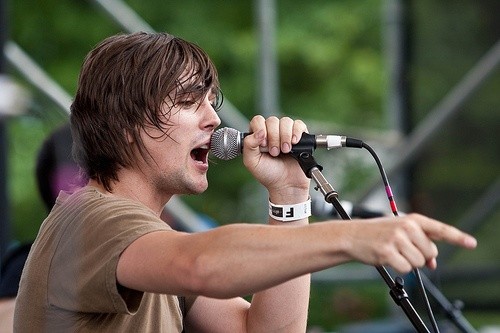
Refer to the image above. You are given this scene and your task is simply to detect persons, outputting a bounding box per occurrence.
[11,28,478,333]
[1,126,87,333]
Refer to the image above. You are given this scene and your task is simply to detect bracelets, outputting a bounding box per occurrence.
[268,195,311,222]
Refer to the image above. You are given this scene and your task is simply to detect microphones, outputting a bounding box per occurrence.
[210,126,364,161]
